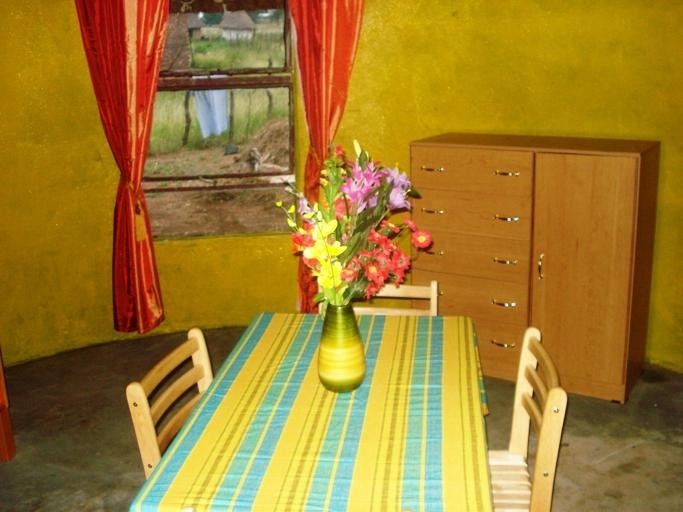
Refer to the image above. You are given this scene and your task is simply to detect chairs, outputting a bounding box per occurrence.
[122,322,219,479]
[486,326,570,512]
[353,280,438,315]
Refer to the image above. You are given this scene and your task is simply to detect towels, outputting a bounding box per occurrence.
[191,74,230,140]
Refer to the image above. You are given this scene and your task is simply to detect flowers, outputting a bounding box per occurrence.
[271,141,435,306]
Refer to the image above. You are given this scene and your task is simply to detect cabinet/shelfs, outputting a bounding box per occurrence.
[406,130,662,407]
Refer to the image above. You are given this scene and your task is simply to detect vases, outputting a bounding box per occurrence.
[314,295,367,393]
